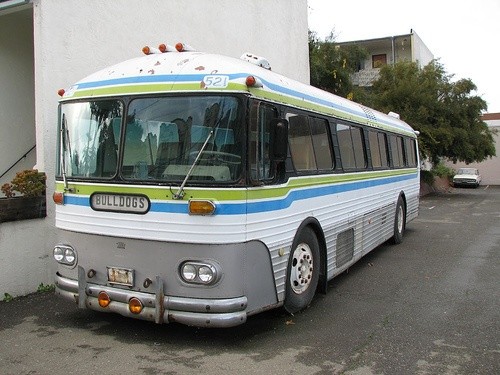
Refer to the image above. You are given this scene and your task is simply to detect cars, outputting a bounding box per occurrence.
[453,168,481,188]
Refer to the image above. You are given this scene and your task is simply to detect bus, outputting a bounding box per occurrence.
[51,40,421,330]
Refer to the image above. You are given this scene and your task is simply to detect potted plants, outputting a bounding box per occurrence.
[11,169,46,217]
[0,183,19,220]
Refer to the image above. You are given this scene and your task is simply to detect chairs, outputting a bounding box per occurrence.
[189,142,217,166]
[153,142,187,179]
[218,144,235,168]
